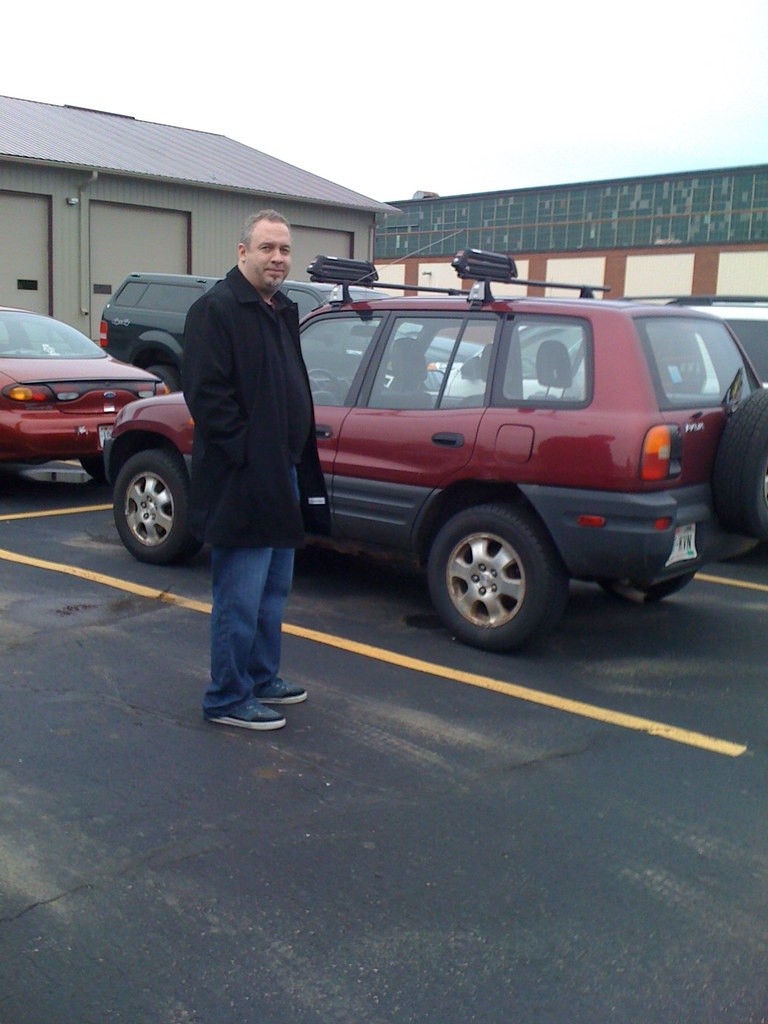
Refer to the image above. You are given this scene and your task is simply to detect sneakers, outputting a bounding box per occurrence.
[204,677,308,731]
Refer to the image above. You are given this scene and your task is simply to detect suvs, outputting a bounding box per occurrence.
[100,271,467,397]
[103,248,768,652]
[439,294,768,419]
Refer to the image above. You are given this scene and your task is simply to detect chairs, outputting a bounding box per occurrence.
[528,340,574,400]
[460,343,513,406]
[389,336,432,408]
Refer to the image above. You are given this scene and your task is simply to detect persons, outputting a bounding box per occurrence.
[182,209,331,730]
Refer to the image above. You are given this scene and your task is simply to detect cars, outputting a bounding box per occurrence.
[0,305,169,488]
